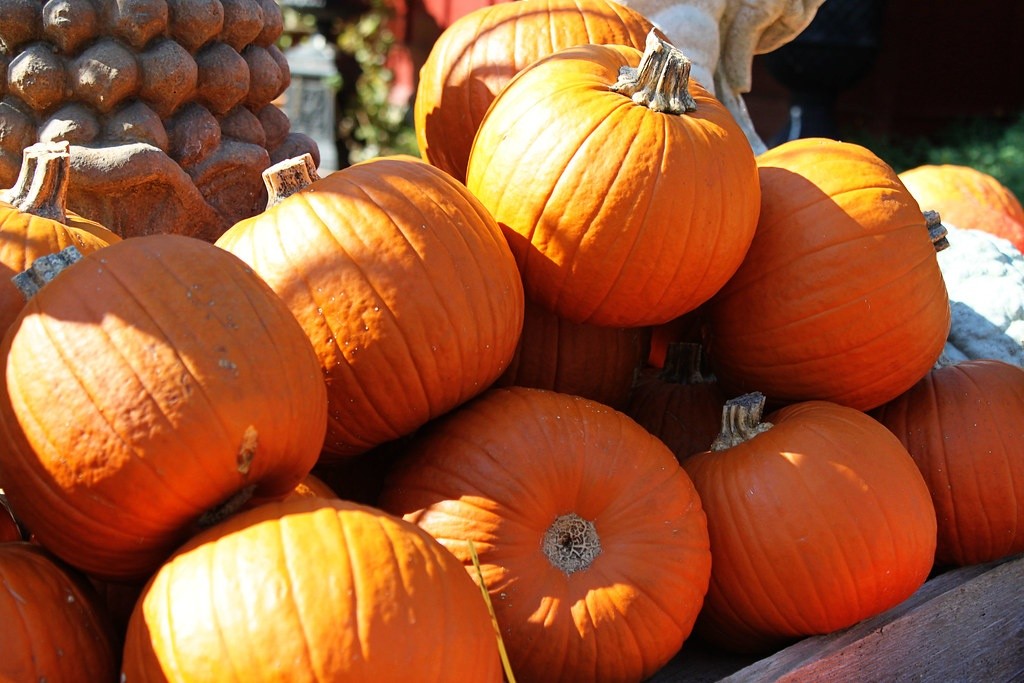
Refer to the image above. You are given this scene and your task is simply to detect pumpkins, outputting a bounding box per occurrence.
[0,0,1024,682]
